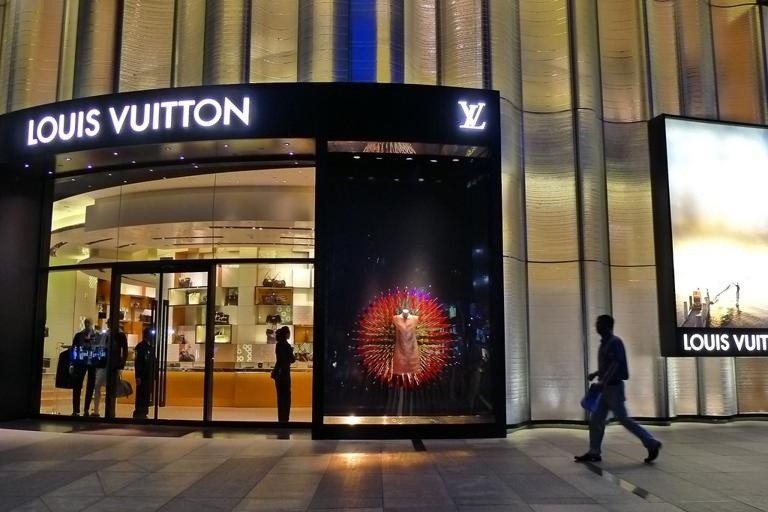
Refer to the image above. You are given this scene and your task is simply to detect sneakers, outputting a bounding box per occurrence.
[575,453,602,461]
[644,441,662,462]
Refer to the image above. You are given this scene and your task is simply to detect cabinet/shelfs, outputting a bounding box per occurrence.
[167,287,314,370]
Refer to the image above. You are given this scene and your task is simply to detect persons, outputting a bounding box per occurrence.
[573,315,663,464]
[271,326,296,439]
[57,318,156,421]
[392,308,421,376]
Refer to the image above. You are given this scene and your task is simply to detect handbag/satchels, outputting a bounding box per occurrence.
[271,367,283,379]
[116,379,133,397]
